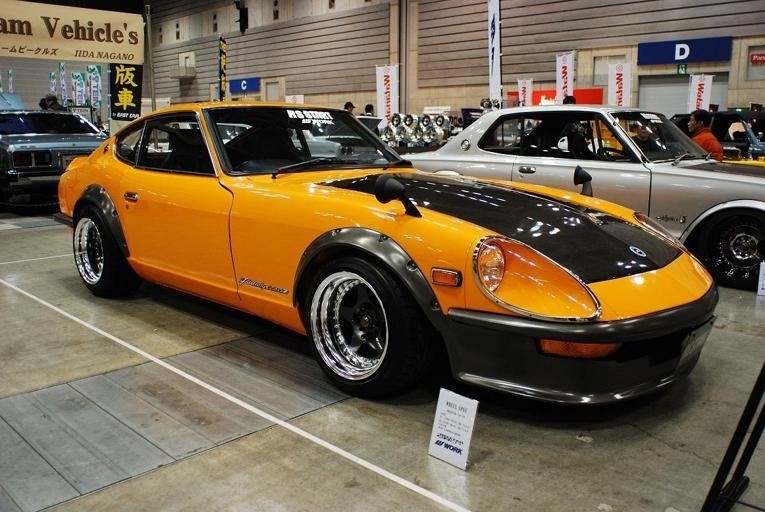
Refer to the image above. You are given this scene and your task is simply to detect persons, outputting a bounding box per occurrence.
[687,109,723,162]
[623,118,658,162]
[480,98,492,116]
[39,98,47,110]
[364,104,375,116]
[554,95,590,149]
[46,93,64,112]
[343,102,356,112]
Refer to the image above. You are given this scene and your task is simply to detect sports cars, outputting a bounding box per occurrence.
[55,100,721,405]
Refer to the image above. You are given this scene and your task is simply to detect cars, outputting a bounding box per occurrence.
[0,90,113,203]
[382,106,765,286]
[176,112,389,163]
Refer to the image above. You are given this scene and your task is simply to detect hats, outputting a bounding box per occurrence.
[345,102,356,108]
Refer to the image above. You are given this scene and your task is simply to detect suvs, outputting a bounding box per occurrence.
[669,110,765,156]
